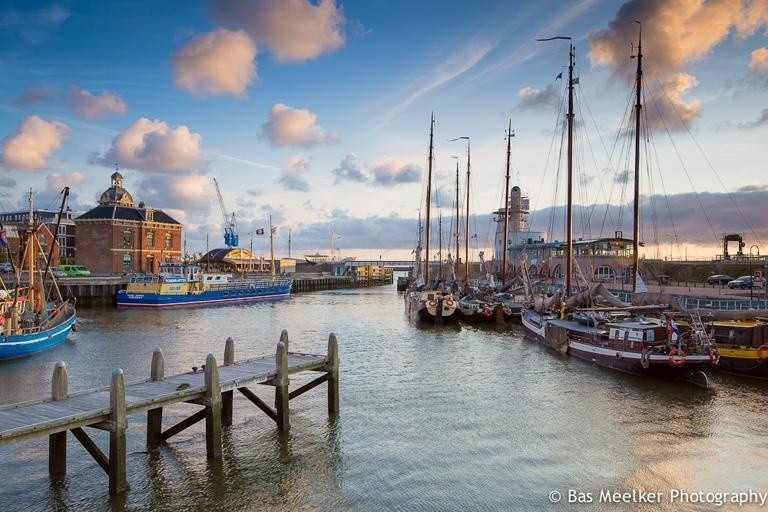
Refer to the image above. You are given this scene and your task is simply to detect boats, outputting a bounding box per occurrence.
[115,261,295,311]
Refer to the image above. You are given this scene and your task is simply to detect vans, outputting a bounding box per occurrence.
[58,264,92,277]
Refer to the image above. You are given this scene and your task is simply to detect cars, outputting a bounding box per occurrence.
[43,266,67,278]
[0,261,17,274]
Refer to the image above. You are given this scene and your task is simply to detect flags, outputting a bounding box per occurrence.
[665,314,681,341]
[255,228,264,234]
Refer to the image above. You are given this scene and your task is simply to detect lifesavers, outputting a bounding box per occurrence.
[505,308,512,314]
[710,349,720,365]
[524,301,530,309]
[446,299,453,306]
[669,349,686,366]
[482,307,491,316]
[429,300,436,306]
[757,345,768,360]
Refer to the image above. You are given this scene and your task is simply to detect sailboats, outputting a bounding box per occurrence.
[0,179,78,356]
